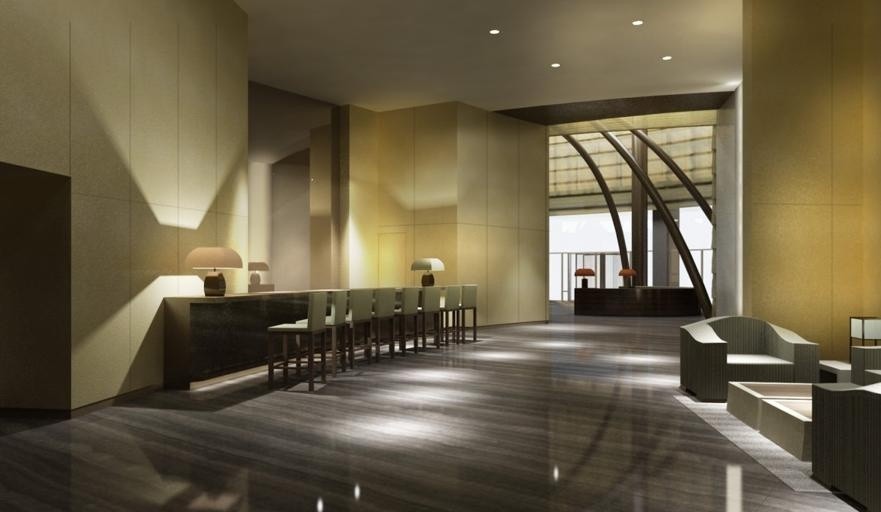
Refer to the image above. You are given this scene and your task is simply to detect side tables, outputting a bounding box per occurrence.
[819,360,852,384]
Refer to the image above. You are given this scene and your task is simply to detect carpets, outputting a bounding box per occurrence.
[672,393,842,493]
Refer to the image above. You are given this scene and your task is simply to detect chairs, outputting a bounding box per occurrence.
[267,285,478,392]
[680,316,819,402]
[849,346,881,386]
[812,381,881,512]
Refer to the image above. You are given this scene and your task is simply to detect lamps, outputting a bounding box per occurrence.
[849,317,881,363]
[411,258,445,286]
[184,247,242,296]
[575,269,594,289]
[619,269,637,287]
